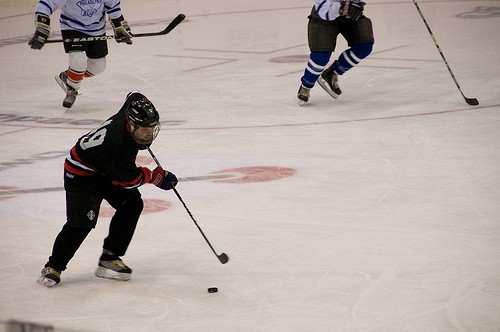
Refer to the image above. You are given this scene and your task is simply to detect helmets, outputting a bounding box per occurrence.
[124,91,161,145]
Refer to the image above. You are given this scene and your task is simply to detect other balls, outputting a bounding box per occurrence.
[207,287,218,293]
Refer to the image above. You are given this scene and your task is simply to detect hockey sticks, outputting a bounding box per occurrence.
[147,146,229,264]
[45,13,186,43]
[412,0,479,106]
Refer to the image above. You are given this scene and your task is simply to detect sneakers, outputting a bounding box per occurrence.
[316,64,343,99]
[62,84,82,113]
[36,267,61,287]
[297,84,311,106]
[54,71,69,94]
[95,248,132,281]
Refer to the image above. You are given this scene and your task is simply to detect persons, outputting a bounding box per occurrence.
[28,0,133,113]
[296,0,374,106]
[36,91,178,287]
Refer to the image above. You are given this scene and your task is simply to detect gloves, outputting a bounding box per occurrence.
[28,14,51,50]
[148,166,178,190]
[108,14,134,45]
[139,142,152,150]
[347,0,366,21]
